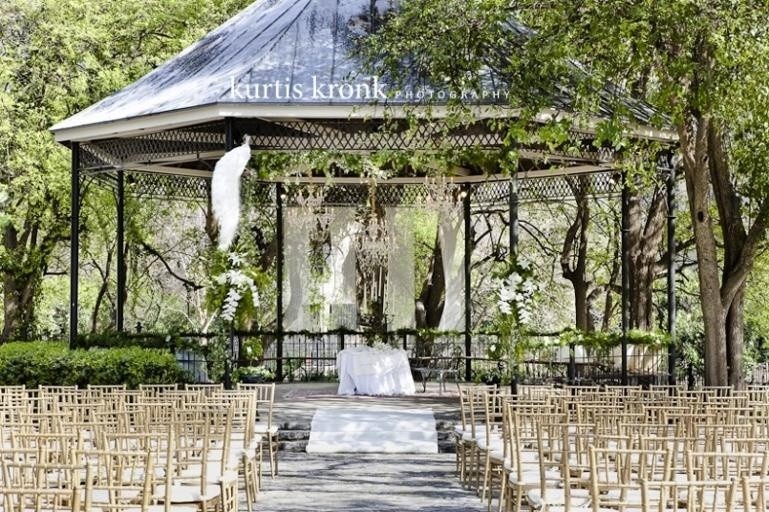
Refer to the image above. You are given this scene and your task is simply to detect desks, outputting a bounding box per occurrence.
[340,349,407,395]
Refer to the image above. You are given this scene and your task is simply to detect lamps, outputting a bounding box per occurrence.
[354,177,397,268]
[297,169,336,242]
[416,170,462,215]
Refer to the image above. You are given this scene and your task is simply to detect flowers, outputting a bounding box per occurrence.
[489,252,549,387]
[206,239,271,324]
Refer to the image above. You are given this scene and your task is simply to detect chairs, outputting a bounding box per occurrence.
[412,342,461,395]
[1,381,280,512]
[452,382,769,510]
